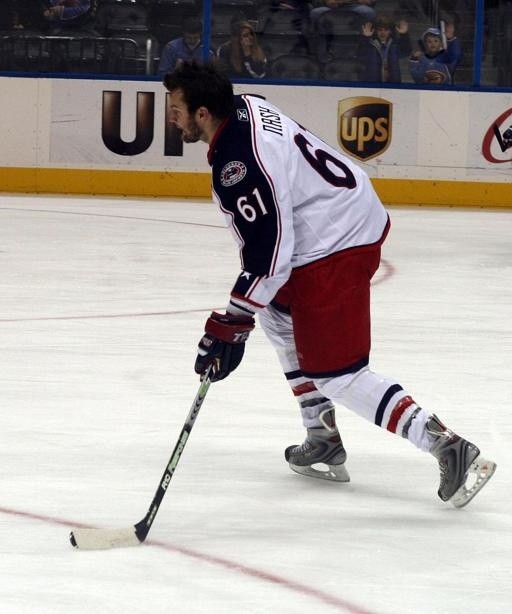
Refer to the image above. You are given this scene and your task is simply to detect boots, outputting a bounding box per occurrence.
[285,406,347,466]
[424,413,480,502]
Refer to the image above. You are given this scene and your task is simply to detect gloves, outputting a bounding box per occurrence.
[194,311,255,382]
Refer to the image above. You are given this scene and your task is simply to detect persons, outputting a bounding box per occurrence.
[1,0,461,85]
[164,56,497,509]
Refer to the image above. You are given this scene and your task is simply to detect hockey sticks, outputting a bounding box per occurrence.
[69,361,221,551]
